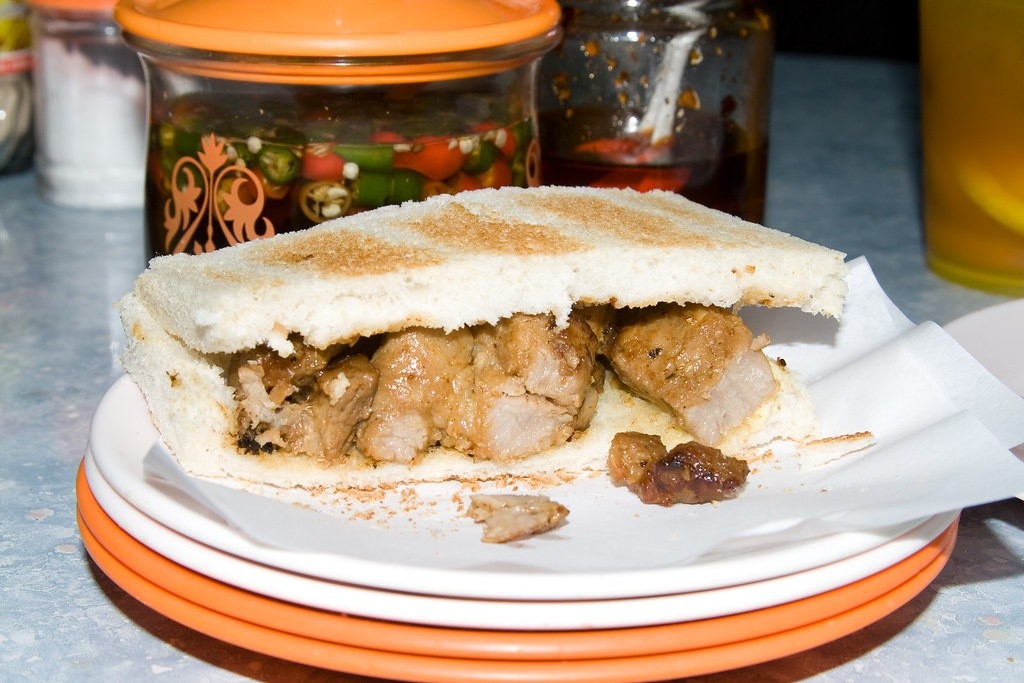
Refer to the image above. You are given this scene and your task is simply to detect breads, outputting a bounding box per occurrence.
[106,185,853,490]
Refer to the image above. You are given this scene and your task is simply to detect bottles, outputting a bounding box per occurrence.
[112,1,563,268]
[27,0,147,212]
[541,0,777,238]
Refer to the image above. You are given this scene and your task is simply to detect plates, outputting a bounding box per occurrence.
[73,373,964,683]
[941,300,1024,501]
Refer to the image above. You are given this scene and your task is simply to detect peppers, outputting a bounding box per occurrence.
[157,90,536,225]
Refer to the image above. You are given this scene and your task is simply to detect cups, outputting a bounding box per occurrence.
[918,0,1024,298]
